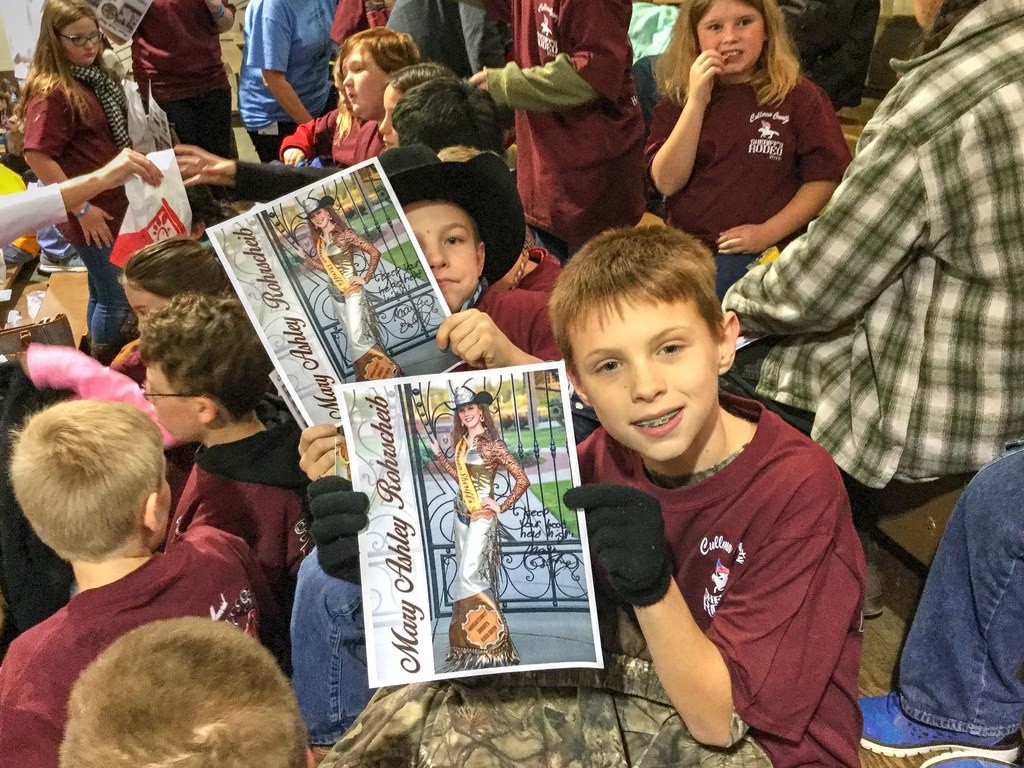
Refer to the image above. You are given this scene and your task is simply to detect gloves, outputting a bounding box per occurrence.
[308,477,370,584]
[564,483,672,605]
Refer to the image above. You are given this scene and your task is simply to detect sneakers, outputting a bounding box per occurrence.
[918,752,1022,768]
[858,694,1024,761]
[40,251,89,272]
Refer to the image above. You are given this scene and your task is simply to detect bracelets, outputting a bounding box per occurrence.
[211,6,224,20]
[74,203,90,218]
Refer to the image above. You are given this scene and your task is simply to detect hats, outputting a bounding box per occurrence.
[442,387,494,412]
[380,144,526,285]
[297,196,335,219]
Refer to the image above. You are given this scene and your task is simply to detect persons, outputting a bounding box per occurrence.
[429,386,530,670]
[715,0,1024,508]
[0,0,849,768]
[294,196,407,382]
[854,448,1024,768]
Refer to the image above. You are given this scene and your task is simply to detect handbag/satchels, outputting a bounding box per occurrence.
[0,314,76,362]
[108,148,192,270]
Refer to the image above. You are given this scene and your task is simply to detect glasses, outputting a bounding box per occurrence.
[143,387,212,406]
[59,32,102,46]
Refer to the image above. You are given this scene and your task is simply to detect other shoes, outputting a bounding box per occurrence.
[861,555,883,617]
